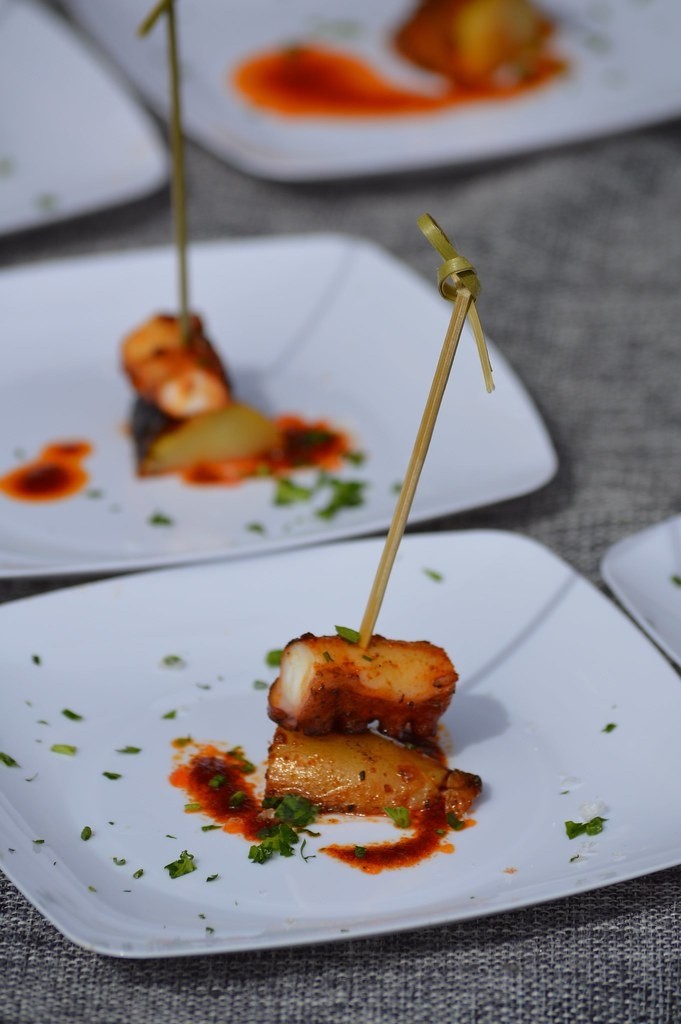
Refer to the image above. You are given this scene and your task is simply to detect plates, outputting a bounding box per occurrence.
[0,2,169,237]
[0,230,559,580]
[0,529,681,960]
[601,517,681,667]
[63,0,681,184]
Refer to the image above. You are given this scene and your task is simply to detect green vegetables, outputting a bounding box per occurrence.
[0,623,610,883]
[142,442,371,536]
